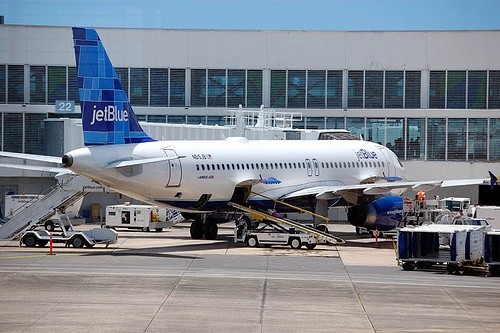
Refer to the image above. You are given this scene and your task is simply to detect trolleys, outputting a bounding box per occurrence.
[396,219,493,271]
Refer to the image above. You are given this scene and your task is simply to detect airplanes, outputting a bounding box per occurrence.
[0,27,500,245]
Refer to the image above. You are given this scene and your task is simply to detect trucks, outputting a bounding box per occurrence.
[234,213,326,249]
[19,224,118,247]
[100,202,173,232]
[40,216,86,231]
